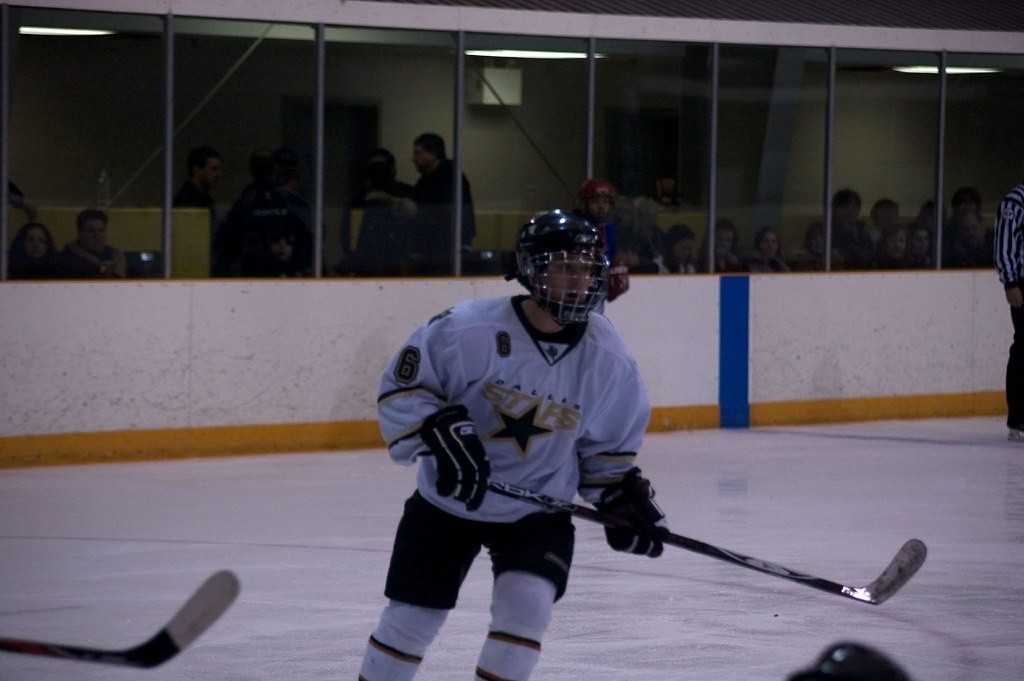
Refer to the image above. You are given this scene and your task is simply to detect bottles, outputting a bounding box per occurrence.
[96,169,111,208]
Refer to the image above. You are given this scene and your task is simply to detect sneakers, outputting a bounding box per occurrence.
[1008,428,1024,442]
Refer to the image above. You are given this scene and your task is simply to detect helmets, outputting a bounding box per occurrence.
[512,209,608,326]
[784,639,914,681]
[578,177,616,201]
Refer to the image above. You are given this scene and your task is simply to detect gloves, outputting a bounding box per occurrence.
[419,404,492,512]
[593,467,670,558]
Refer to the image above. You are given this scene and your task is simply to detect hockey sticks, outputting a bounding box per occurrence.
[485,480,928,607]
[0,569,241,670]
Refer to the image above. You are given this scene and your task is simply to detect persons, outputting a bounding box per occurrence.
[8,131,990,282]
[356,211,667,681]
[996,183,1024,440]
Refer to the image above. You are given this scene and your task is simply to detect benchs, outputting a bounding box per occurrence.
[7,206,210,278]
[351,207,998,276]
